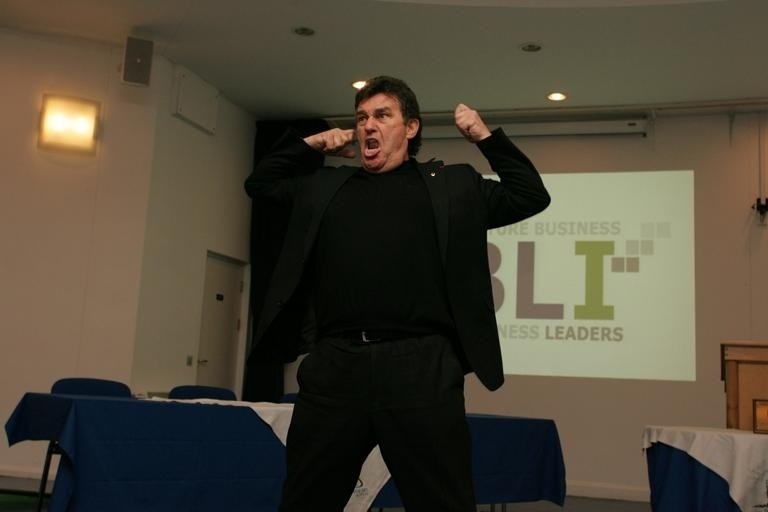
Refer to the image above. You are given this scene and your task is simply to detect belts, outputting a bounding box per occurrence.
[350,330,384,343]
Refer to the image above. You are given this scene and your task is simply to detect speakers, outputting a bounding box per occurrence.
[120,34,154,88]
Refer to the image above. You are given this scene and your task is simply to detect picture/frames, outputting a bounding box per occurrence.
[752,399,768,434]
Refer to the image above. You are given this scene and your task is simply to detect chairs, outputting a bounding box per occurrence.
[280,394,297,403]
[36,377,133,512]
[169,386,237,401]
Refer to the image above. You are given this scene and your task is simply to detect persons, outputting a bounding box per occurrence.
[244,75,551,512]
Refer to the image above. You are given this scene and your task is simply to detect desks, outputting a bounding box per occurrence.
[5,393,566,512]
[640,425,768,512]
